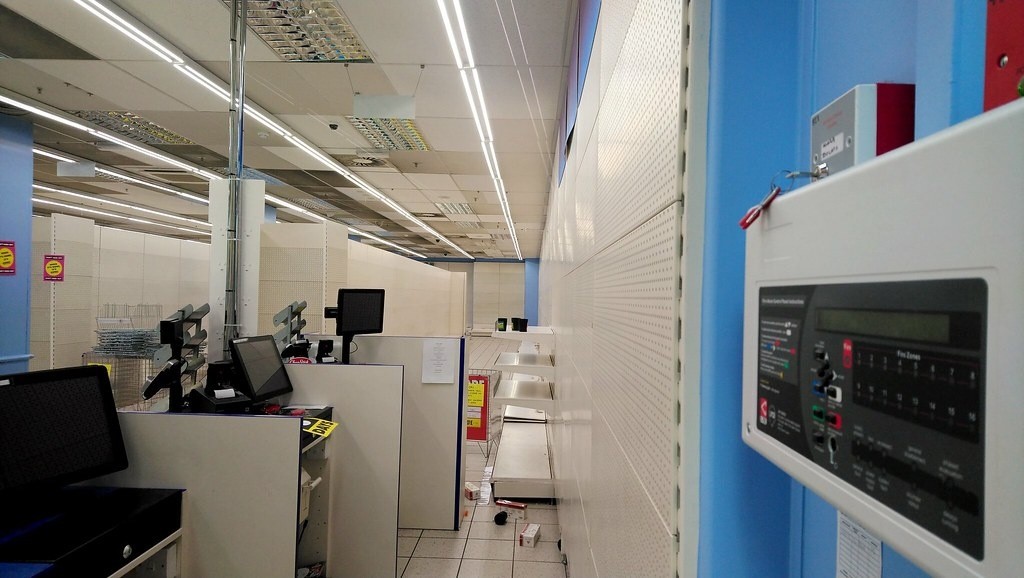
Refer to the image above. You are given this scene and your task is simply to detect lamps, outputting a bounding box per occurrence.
[0,0,524,260]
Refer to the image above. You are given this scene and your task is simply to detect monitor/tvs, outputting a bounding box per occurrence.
[336,288,385,335]
[0,363,129,509]
[229,334,294,402]
